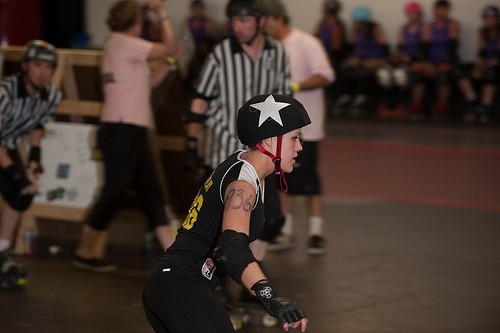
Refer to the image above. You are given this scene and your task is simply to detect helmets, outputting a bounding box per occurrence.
[224,0,267,16]
[236,93,312,144]
[23,39,58,63]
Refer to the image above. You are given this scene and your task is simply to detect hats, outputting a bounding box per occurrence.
[405,1,423,13]
[352,6,372,20]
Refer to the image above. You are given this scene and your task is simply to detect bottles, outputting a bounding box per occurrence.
[24,228,32,257]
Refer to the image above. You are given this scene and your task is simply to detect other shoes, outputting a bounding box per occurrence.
[0,251,28,288]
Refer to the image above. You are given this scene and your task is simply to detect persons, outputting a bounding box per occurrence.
[71,0,180,272]
[255,0,335,256]
[142,0,500,124]
[0,41,70,282]
[190,0,294,312]
[142,94,313,333]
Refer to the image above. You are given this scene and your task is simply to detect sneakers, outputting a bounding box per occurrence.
[69,251,115,271]
[306,235,327,254]
[268,234,295,249]
